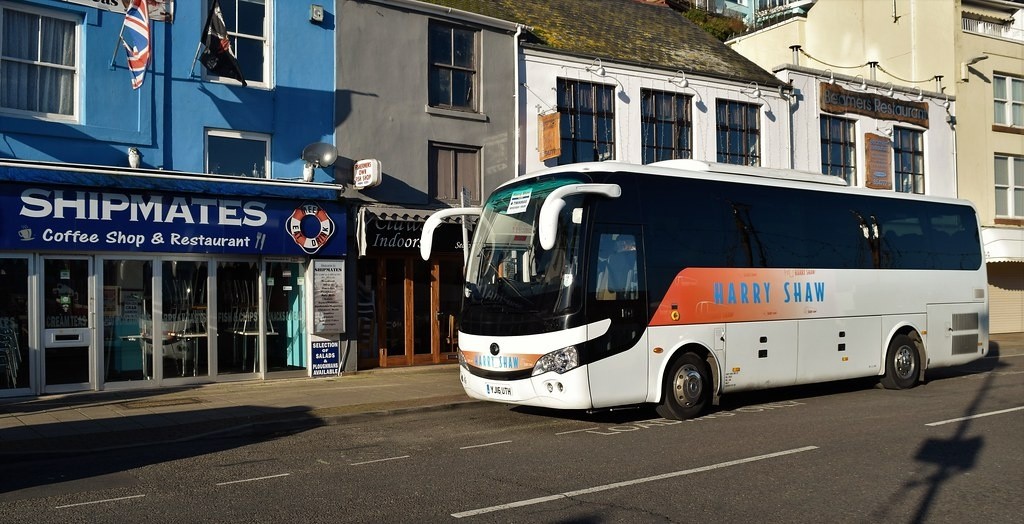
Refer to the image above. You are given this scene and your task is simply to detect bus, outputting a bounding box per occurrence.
[422,158,992,419]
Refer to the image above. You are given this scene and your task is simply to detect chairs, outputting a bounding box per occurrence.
[596,255,616,300]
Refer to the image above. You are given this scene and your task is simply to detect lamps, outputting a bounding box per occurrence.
[849,74,867,90]
[739,81,761,98]
[586,57,605,76]
[878,82,894,97]
[667,70,689,88]
[905,86,924,101]
[936,92,950,109]
[817,69,835,85]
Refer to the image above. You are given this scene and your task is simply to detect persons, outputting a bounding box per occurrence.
[606,234,639,300]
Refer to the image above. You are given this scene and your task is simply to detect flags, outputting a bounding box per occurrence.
[200,0,249,89]
[118,0,153,91]
